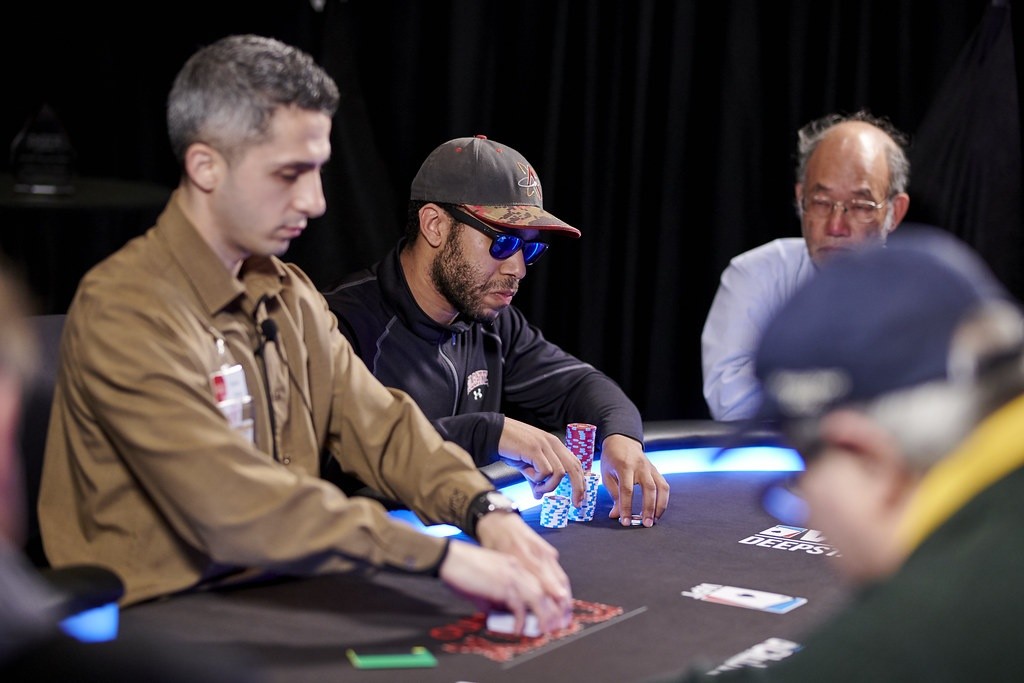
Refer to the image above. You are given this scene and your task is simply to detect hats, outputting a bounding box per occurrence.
[411,135,581,238]
[711,229,1024,459]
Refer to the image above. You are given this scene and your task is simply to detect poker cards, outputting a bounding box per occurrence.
[486,609,543,639]
[759,526,800,537]
[708,585,779,609]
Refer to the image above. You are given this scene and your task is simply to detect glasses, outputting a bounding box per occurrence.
[801,189,902,223]
[755,439,825,528]
[443,204,550,266]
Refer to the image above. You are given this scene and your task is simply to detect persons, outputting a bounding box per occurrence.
[36,34,571,639]
[0,272,89,683]
[700,121,910,421]
[712,249,1024,682]
[319,136,670,527]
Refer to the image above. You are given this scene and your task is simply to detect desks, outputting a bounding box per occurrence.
[118,416,874,682]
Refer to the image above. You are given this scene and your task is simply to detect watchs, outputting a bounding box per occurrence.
[467,490,520,536]
